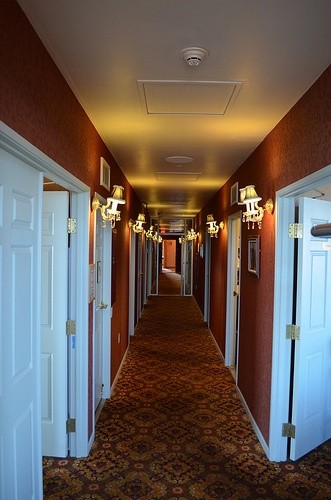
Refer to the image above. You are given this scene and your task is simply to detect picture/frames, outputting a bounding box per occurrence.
[247,235,260,279]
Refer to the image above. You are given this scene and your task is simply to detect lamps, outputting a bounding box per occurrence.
[129,213,147,233]
[238,185,274,230]
[144,226,163,243]
[179,228,199,243]
[206,214,224,238]
[92,184,126,229]
